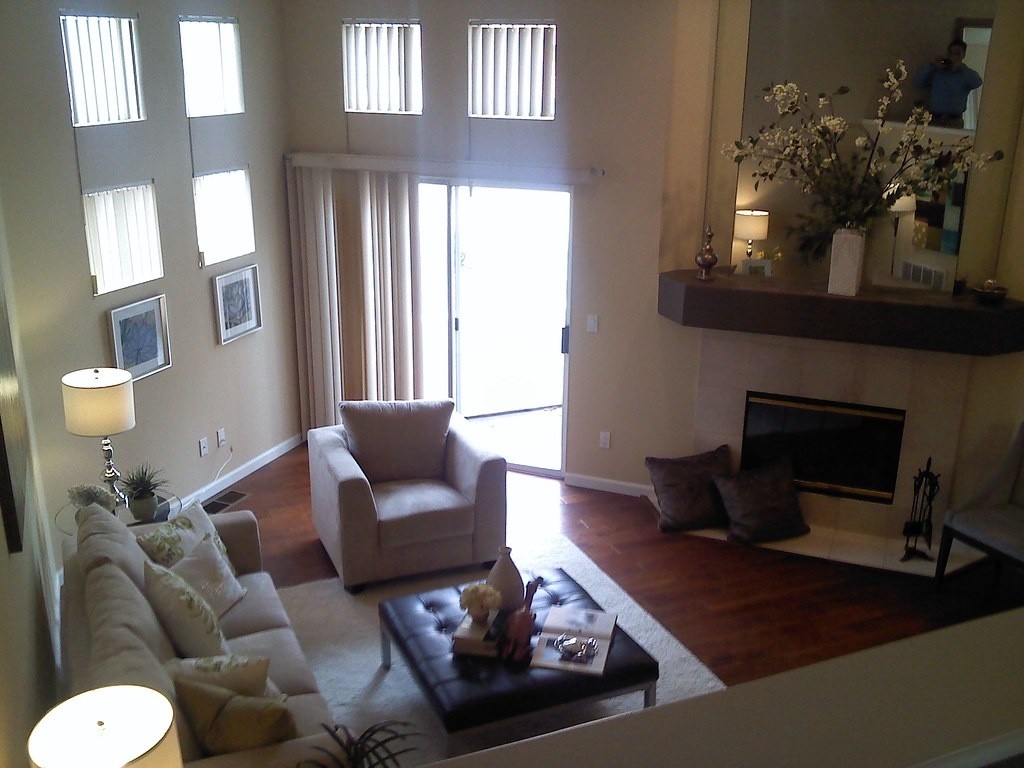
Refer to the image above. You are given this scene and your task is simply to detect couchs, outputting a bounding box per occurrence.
[305,397,507,584]
[60,502,361,768]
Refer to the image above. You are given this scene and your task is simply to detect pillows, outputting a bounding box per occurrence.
[173,677,306,757]
[142,559,230,657]
[165,654,290,704]
[135,498,227,569]
[645,443,731,536]
[711,453,812,545]
[166,532,248,618]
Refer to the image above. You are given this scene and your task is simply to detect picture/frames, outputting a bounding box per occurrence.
[211,262,264,347]
[107,292,173,383]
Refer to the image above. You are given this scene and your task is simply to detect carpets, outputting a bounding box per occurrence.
[273,533,727,768]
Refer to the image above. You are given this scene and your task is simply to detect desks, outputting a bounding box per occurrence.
[377,565,659,757]
[53,486,183,538]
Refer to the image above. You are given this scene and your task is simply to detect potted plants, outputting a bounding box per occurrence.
[117,461,170,521]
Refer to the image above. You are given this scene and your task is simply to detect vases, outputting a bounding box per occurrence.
[470,609,489,624]
[828,228,867,297]
[486,547,525,614]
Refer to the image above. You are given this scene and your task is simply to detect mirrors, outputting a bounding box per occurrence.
[728,0,1000,292]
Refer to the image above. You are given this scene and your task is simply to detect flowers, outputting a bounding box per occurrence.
[712,55,1004,222]
[460,583,503,615]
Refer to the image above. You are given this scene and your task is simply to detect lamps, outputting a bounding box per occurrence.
[735,208,769,257]
[26,684,184,768]
[61,366,136,507]
[883,182,916,276]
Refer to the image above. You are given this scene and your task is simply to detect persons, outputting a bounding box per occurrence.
[913,39,983,129]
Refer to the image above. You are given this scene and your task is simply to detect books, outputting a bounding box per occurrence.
[529,605,618,676]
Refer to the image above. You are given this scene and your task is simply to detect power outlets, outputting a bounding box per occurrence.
[199,436,208,458]
[217,428,226,448]
[600,431,611,449]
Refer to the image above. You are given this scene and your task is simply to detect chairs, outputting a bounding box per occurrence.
[927,418,1024,610]
